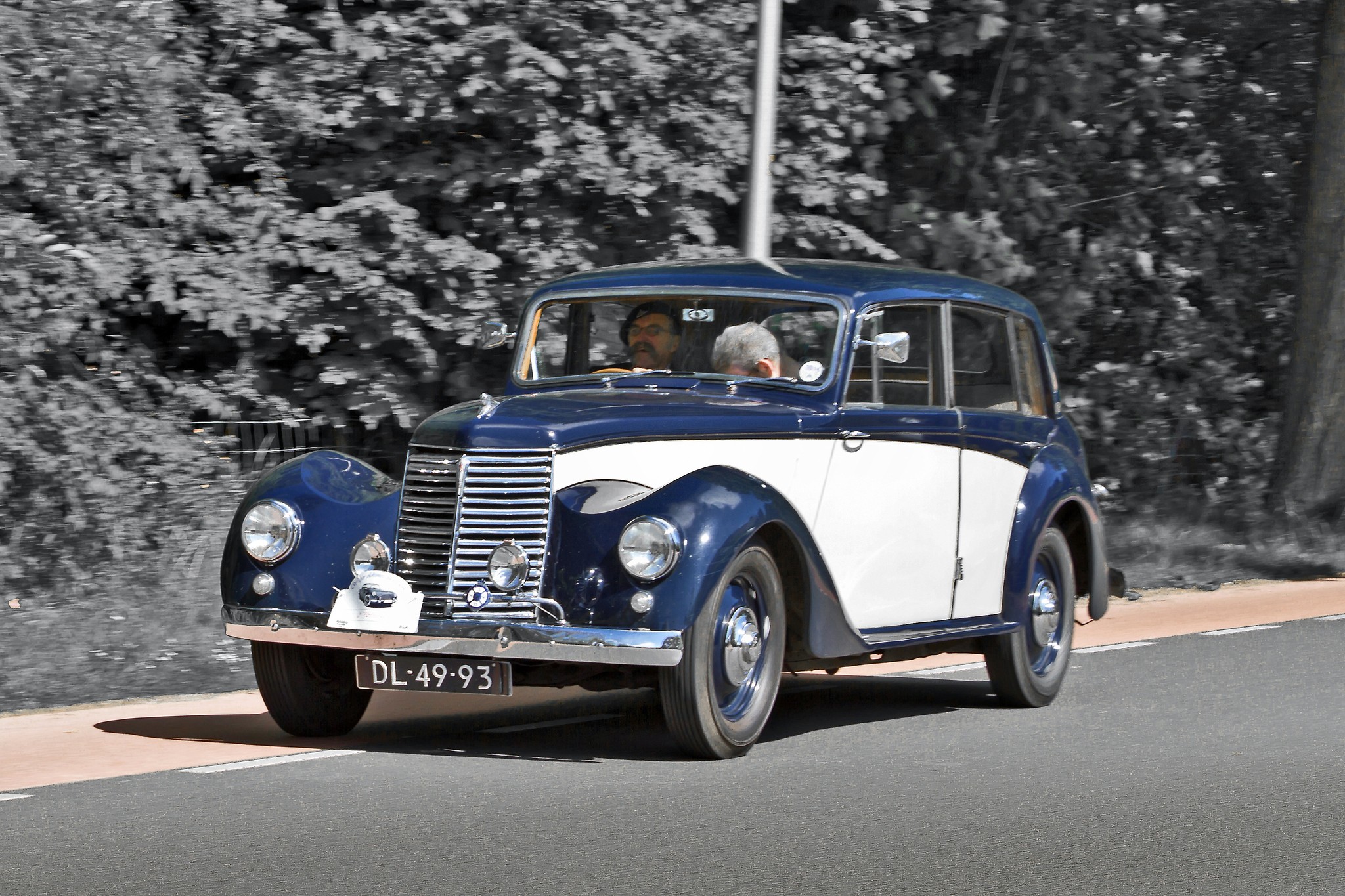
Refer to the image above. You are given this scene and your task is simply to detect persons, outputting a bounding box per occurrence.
[711,321,803,380]
[588,302,693,372]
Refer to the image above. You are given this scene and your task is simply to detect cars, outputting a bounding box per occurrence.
[221,256,1109,760]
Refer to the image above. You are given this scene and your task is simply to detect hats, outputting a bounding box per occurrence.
[620,303,683,345]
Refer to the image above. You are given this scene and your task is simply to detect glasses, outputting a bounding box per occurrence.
[629,325,677,337]
